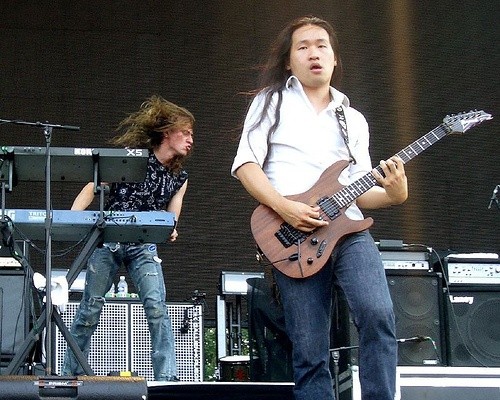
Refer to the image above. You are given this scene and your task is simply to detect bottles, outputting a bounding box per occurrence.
[108,282,115,297]
[117,275,128,298]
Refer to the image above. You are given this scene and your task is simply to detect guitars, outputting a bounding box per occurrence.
[250,108,494,279]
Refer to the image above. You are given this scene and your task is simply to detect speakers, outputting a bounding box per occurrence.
[51,296,203,382]
[340,269,447,367]
[441,285,500,367]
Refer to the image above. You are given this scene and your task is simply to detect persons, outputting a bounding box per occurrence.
[62,97,195,382]
[231,17,408,400]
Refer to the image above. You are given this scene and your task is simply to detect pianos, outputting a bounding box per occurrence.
[0,119,177,383]
